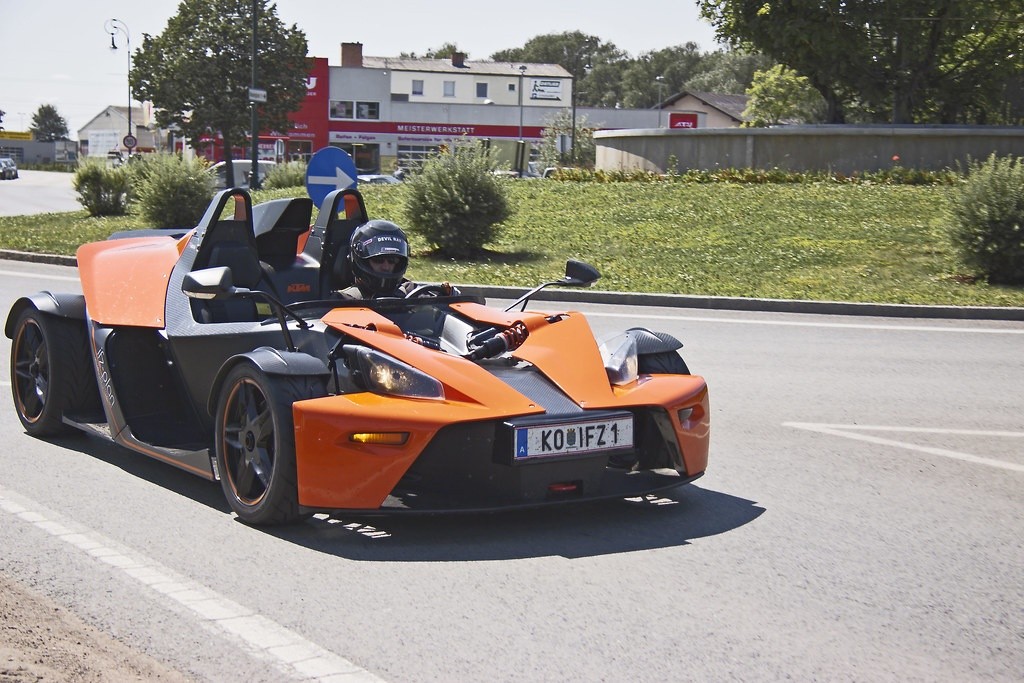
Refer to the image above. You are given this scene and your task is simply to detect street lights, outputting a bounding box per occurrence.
[104,16,132,157]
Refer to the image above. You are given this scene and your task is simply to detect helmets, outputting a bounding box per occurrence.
[349,219,411,293]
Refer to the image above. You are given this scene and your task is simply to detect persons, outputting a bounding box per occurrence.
[330,219,461,335]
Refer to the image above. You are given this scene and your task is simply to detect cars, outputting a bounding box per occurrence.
[491,167,580,178]
[0,158,18,180]
[356,167,422,183]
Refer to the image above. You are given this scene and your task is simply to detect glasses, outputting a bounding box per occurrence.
[371,254,399,264]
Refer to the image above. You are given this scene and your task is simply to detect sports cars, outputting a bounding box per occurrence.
[4,183,713,524]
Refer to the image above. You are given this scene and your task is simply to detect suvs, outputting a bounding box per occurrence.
[204,160,279,189]
